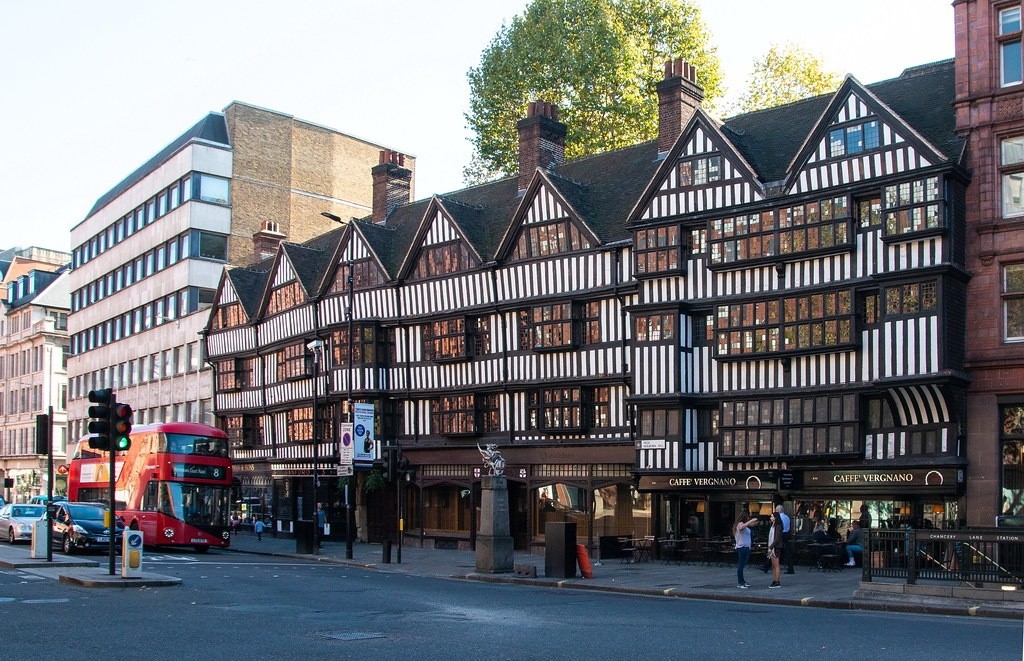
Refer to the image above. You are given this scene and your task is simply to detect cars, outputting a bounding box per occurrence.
[0,495,5,508]
[40,501,127,555]
[0,503,49,545]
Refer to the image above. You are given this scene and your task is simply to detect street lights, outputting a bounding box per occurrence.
[321,210,356,562]
[307,339,323,545]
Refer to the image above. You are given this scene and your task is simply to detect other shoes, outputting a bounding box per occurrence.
[757,566,767,573]
[784,571,794,574]
[768,581,781,588]
[844,562,856,566]
[737,585,748,588]
[745,582,751,587]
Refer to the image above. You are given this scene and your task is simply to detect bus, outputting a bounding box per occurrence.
[68,421,242,552]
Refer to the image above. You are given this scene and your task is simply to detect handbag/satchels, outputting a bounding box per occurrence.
[324,524,331,535]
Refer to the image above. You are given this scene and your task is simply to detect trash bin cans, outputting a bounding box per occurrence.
[122,530,144,579]
[545,522,577,578]
[31,520,47,559]
[296,521,314,554]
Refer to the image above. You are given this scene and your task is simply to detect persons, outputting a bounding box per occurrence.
[254,517,266,542]
[845,521,864,566]
[733,512,760,589]
[766,512,784,588]
[759,505,795,574]
[317,503,328,548]
[0,495,6,509]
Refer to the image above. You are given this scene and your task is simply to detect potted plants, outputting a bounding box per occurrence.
[870,532,887,569]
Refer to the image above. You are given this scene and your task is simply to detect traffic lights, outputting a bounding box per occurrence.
[113,402,132,451]
[88,387,114,450]
[374,447,395,484]
[398,448,416,484]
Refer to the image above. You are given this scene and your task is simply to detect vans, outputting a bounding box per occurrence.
[29,496,68,504]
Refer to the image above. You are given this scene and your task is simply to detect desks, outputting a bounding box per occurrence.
[807,542,835,574]
[706,540,732,569]
[620,538,654,564]
[659,539,689,565]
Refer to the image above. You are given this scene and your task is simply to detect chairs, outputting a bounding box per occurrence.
[618,535,844,573]
